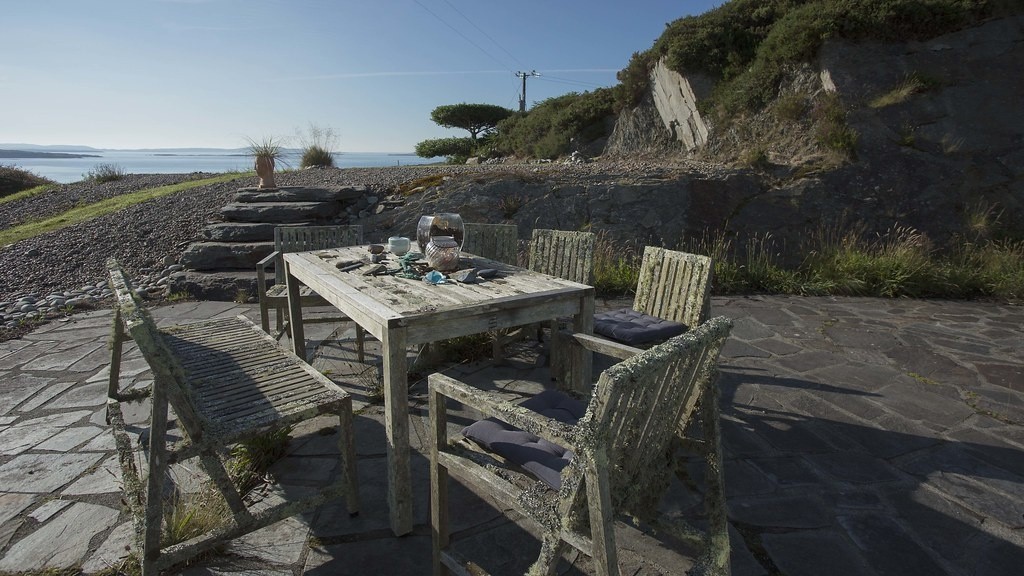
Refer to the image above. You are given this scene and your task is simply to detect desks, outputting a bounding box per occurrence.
[281,240,595,537]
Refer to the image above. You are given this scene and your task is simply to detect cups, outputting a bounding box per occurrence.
[388,236,409,254]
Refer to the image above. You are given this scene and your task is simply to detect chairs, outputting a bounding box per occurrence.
[493,228,595,365]
[590,247,714,363]
[462,221,518,365]
[428,316,736,576]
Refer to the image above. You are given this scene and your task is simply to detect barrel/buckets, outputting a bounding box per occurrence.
[417,213,465,256]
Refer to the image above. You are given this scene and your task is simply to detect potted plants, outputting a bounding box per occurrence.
[250,142,289,187]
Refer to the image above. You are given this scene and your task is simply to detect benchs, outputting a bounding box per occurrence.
[104,256,353,576]
[256,224,365,362]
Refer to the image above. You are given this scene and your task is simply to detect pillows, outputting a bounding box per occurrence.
[594,307,688,345]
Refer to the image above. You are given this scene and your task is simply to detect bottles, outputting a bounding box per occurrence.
[426,236,460,271]
